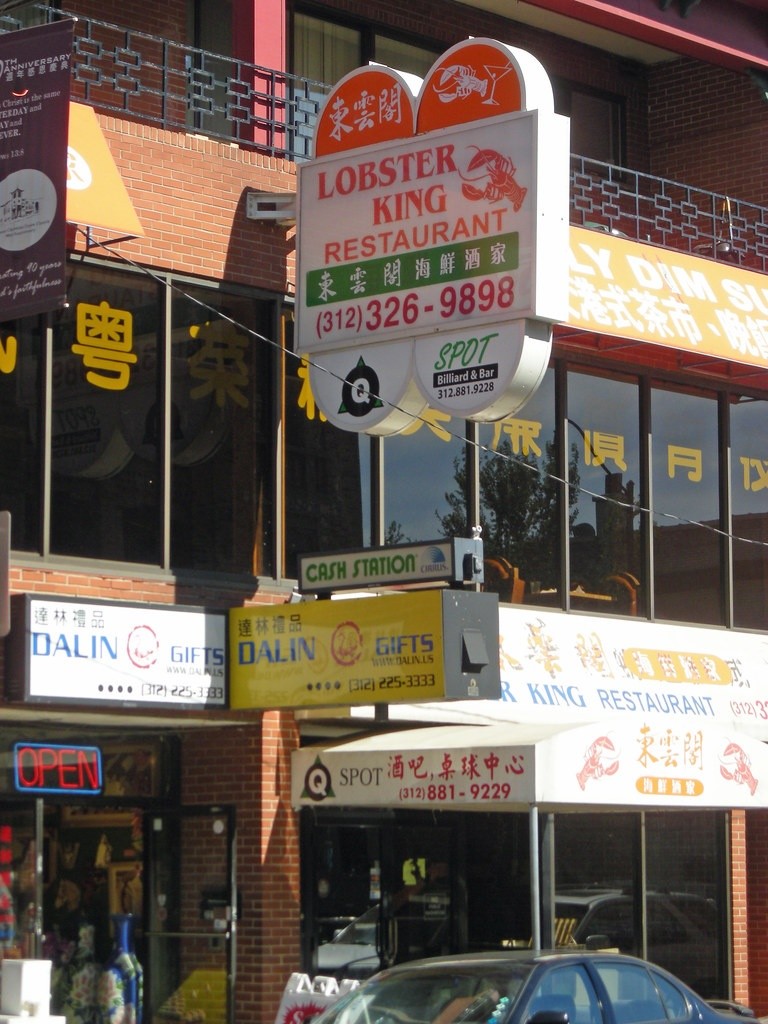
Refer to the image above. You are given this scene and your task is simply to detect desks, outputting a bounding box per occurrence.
[532,588,611,601]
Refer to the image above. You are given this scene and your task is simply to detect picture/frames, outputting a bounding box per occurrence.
[49,810,143,883]
[107,862,143,939]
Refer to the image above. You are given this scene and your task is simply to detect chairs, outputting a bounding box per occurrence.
[484,556,527,603]
[598,572,641,616]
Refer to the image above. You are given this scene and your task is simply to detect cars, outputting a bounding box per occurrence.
[311,946,764,1024]
[558,888,723,1003]
[311,877,509,983]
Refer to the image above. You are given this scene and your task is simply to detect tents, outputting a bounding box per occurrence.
[291,709,768,951]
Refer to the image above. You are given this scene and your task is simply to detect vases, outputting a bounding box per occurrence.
[50,914,143,1024]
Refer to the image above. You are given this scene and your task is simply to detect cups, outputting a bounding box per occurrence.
[530,580,541,593]
